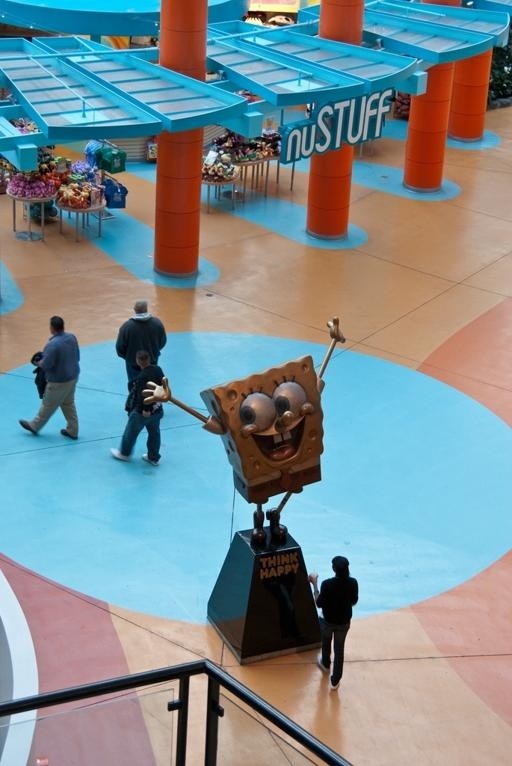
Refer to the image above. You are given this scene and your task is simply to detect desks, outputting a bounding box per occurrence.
[6,188,107,242]
[201,155,295,214]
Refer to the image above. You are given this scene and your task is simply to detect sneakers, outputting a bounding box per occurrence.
[330,672,339,690]
[61,430,77,439]
[142,454,159,465]
[111,448,129,461]
[318,654,328,671]
[20,419,36,433]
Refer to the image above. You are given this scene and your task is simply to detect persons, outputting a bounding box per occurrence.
[308,555,359,691]
[114,297,168,415]
[109,347,166,466]
[18,314,79,439]
